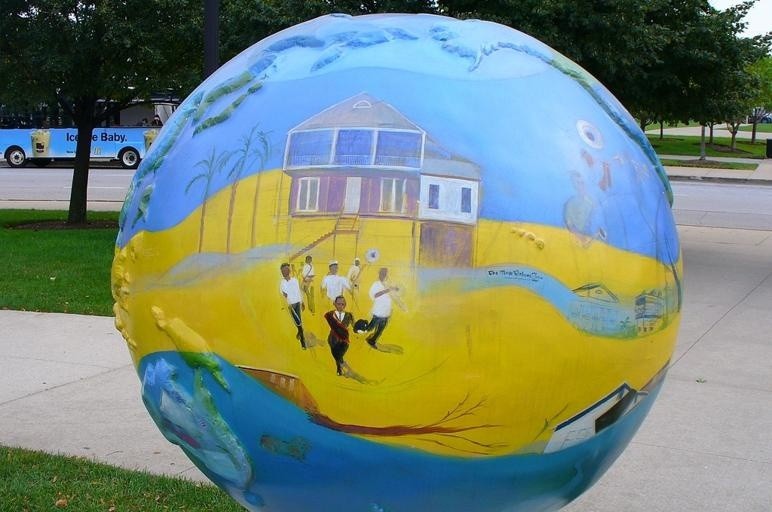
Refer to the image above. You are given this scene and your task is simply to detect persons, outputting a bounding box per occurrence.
[280,255,400,376]
[151,114,163,126]
[140,118,149,126]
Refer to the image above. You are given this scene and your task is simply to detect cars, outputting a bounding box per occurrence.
[740,106,772,123]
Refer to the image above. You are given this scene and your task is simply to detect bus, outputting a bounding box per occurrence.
[0,97,183,169]
